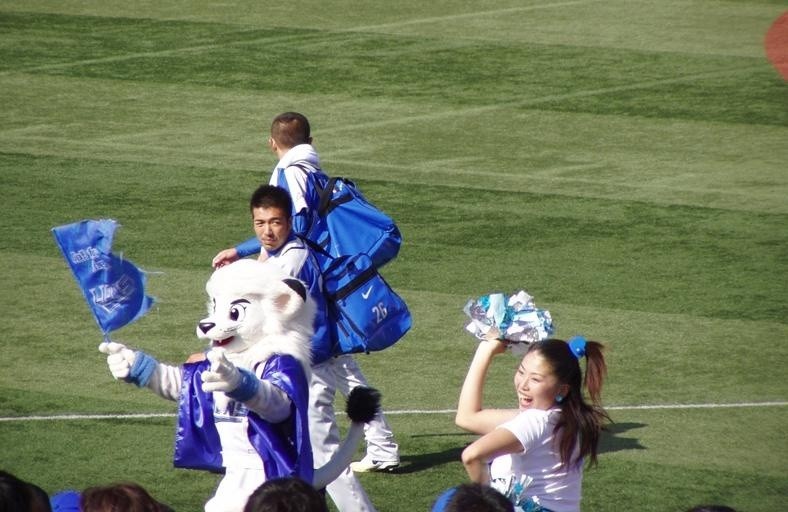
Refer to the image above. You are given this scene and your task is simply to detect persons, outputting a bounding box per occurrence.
[1,469,738,512]
[212,112,401,473]
[250,184,382,512]
[454,338,616,512]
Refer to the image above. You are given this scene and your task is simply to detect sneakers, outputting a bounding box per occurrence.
[350,453,399,473]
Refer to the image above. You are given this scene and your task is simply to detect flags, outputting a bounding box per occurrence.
[51,219,156,343]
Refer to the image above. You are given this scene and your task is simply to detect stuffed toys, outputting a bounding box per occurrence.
[99,260,381,512]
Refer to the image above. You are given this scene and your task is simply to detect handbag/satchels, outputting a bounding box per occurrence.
[320,253,413,358]
[282,163,402,271]
